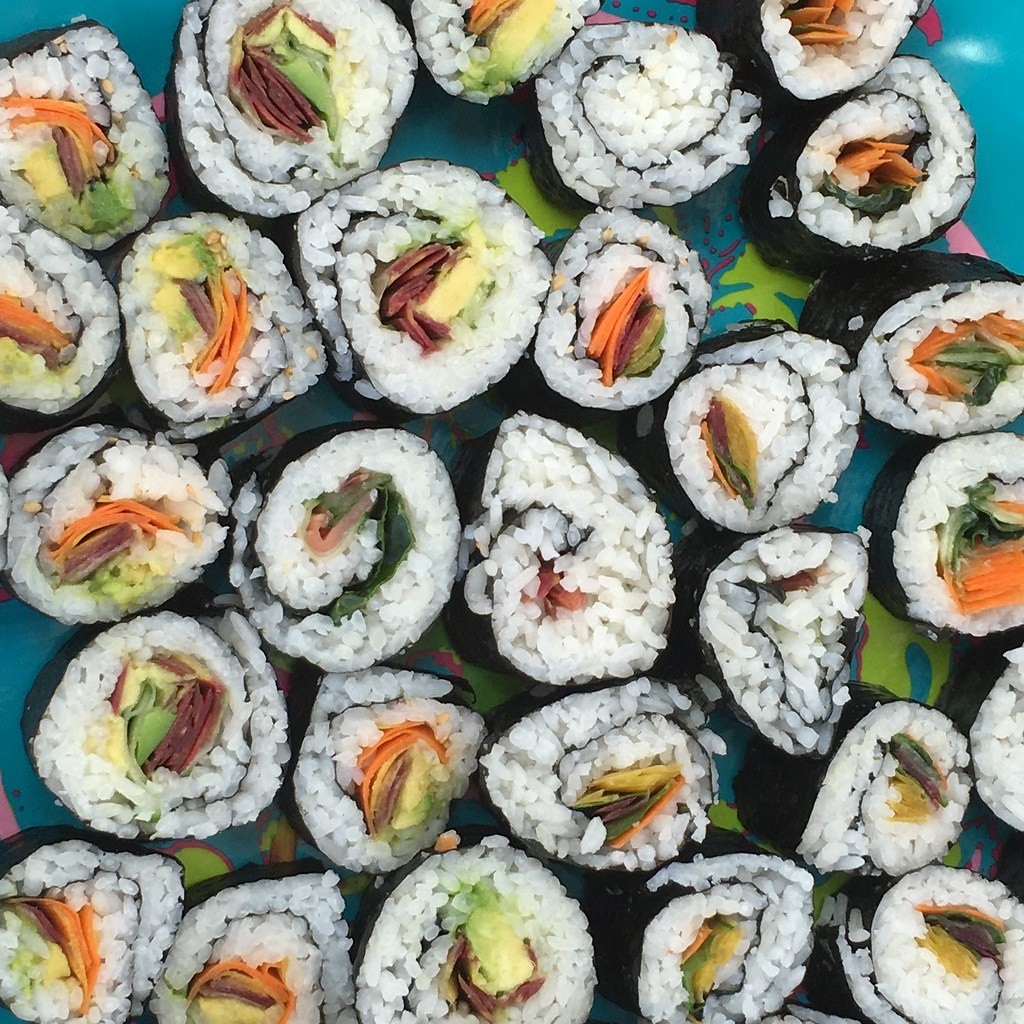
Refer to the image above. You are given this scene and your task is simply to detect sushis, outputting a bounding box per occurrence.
[0,0,1024,1024]
